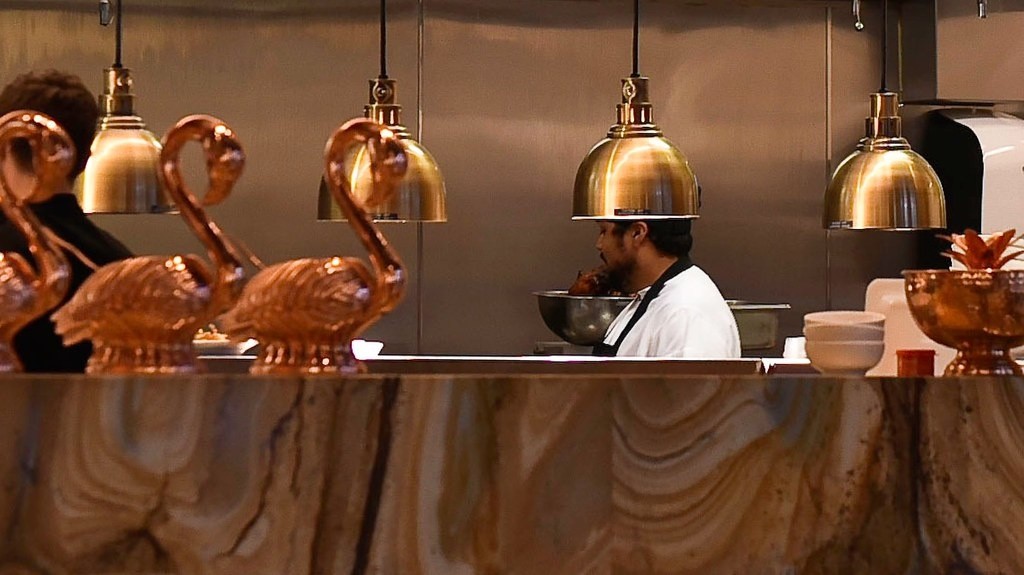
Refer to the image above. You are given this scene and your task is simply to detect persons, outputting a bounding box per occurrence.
[0,66,137,376]
[592,218,742,359]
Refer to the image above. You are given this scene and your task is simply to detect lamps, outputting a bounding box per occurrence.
[572,0,701,221]
[821,0,948,233]
[315,0,449,226]
[79,0,182,215]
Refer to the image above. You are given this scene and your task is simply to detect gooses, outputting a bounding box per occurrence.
[0,108,76,374]
[211,119,409,370]
[52,115,246,369]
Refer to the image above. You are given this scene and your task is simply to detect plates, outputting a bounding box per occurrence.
[193,339,258,356]
[195,356,258,374]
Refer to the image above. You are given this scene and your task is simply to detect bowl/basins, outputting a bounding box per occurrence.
[802,311,886,376]
[533,291,636,348]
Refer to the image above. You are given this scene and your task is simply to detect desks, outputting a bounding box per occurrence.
[0,374,1024,575]
